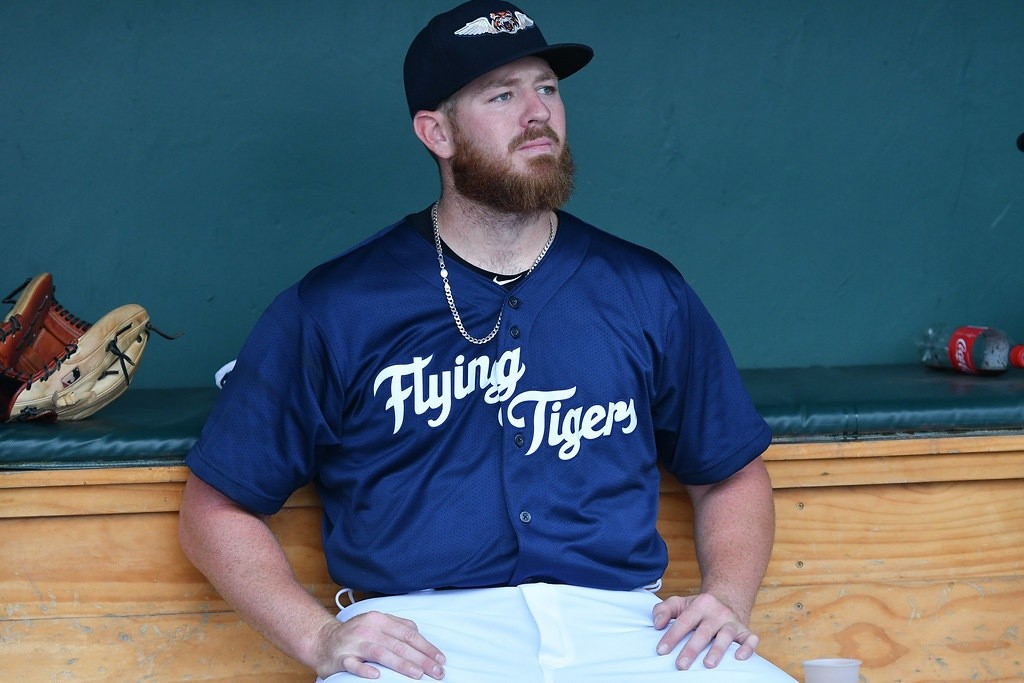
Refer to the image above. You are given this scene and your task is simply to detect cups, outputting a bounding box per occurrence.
[801,658,862,683]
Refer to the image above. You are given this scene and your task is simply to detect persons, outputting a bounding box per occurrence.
[178,0,800,683]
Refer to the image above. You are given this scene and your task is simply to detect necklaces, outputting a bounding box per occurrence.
[430,200,554,344]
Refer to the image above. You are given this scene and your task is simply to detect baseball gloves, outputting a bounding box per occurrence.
[1,271,149,422]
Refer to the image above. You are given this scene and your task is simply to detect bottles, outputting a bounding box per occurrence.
[916,322,1024,377]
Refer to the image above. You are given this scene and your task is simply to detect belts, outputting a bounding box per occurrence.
[363,576,560,599]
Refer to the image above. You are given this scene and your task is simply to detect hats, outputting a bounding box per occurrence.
[403,0,594,122]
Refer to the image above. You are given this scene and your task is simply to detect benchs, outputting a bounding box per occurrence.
[0,363,1024,683]
